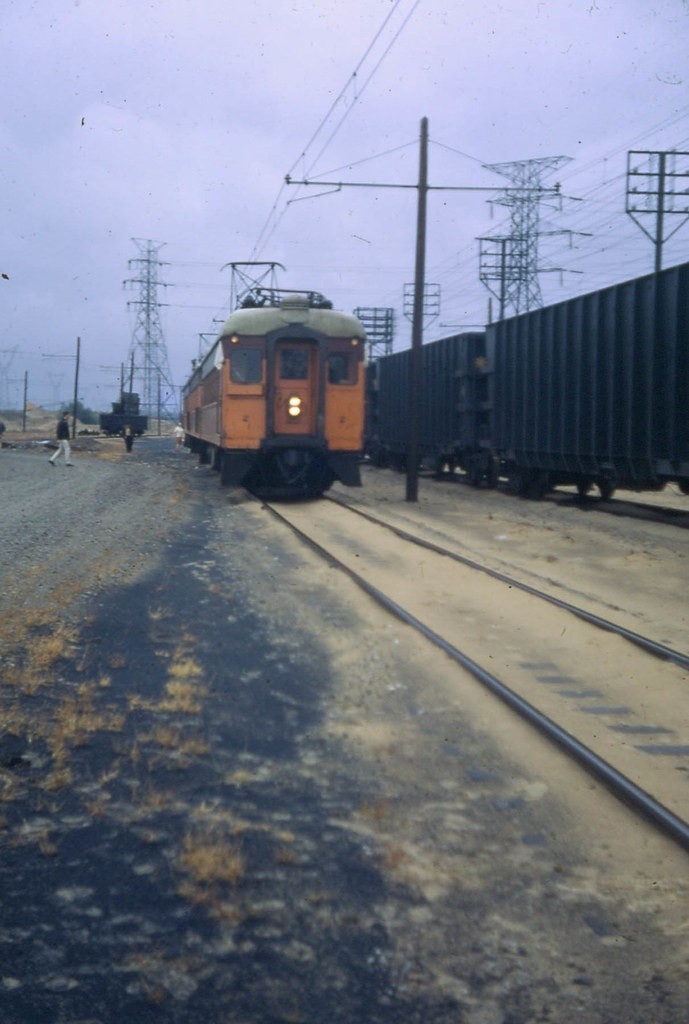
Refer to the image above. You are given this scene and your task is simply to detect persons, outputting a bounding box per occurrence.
[48,411,75,467]
[174,422,185,452]
[122,423,134,453]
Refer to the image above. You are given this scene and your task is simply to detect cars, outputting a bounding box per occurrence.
[78,429,100,435]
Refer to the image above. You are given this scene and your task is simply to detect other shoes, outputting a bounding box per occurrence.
[48,460,55,465]
[66,463,74,467]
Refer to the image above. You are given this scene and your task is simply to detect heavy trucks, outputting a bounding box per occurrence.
[100,413,148,436]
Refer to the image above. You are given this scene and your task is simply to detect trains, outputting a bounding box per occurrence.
[181,295,367,501]
[364,262,689,501]
